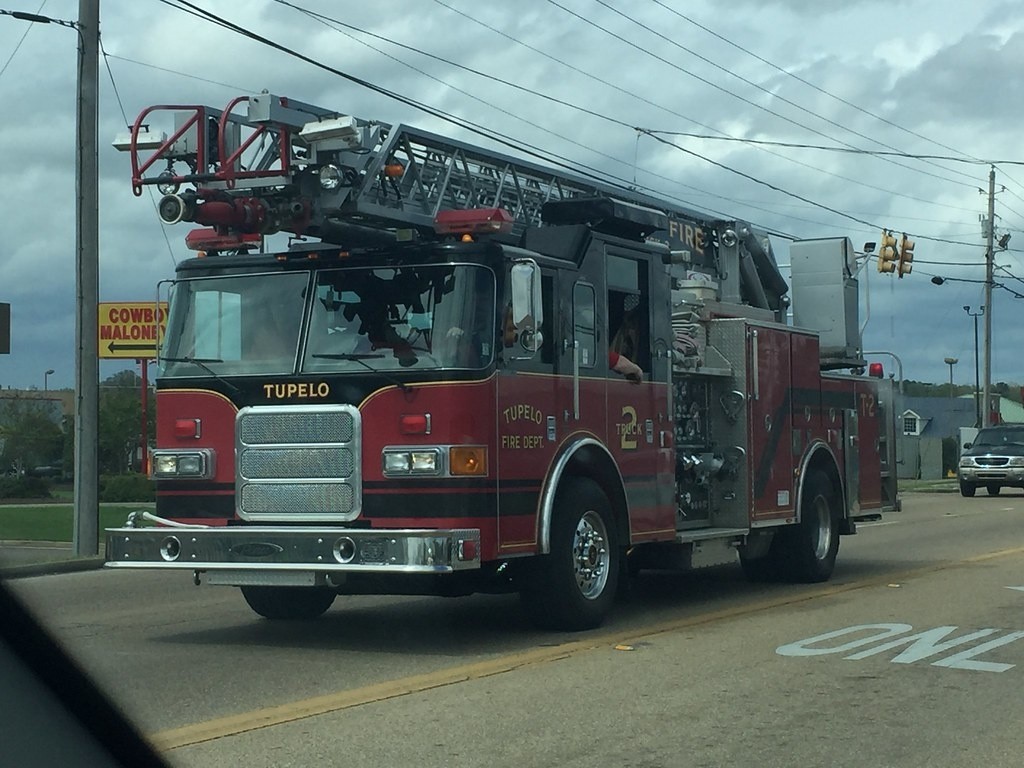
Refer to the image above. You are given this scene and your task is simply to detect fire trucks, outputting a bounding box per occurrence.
[104,88,883,632]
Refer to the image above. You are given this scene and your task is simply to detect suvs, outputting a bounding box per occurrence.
[958,422,1024,498]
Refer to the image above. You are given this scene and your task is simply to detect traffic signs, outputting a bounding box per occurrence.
[99,305,196,361]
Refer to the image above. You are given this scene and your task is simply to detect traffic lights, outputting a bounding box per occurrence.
[897,233,915,280]
[878,230,898,275]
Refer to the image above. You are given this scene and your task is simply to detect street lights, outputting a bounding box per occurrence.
[962,303,986,423]
[944,357,959,398]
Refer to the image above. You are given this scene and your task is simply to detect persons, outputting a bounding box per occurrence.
[610,349,643,382]
[446,305,517,350]
